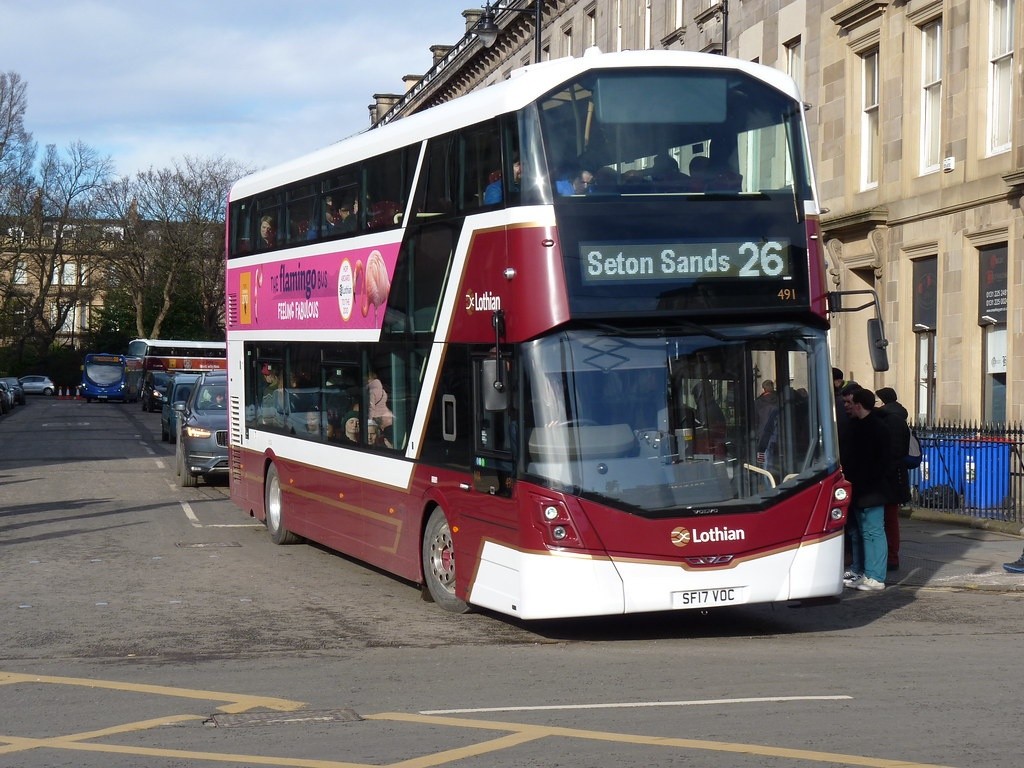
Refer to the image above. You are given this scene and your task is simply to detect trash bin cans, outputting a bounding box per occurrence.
[963,444,1015,509]
[919,441,961,496]
[908,433,977,492]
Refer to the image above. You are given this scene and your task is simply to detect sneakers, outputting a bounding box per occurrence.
[858,578,885,590]
[846,575,866,589]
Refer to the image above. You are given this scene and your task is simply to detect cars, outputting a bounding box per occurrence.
[0,377,26,414]
[19,375,57,395]
[266,386,355,440]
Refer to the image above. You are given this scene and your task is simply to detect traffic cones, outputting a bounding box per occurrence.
[65,386,71,400]
[56,389,64,400]
[76,387,81,400]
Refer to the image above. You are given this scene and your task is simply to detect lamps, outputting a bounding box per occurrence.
[982,315,997,324]
[915,323,930,330]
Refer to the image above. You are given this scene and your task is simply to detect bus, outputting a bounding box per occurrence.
[126,339,226,399]
[224,45,890,622]
[82,353,127,403]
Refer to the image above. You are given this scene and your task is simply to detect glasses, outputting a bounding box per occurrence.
[340,209,351,214]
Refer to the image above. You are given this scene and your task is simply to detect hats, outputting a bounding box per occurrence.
[261,364,273,375]
[368,419,378,427]
[875,387,898,405]
[343,411,359,424]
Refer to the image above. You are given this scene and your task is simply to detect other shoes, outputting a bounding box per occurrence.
[1003,549,1024,572]
[843,574,855,579]
[843,573,861,583]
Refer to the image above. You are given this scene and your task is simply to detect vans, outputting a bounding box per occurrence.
[142,369,170,413]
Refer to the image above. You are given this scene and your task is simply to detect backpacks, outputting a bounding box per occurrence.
[910,428,922,470]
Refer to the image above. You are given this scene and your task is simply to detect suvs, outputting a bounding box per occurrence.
[175,370,229,487]
[161,371,204,444]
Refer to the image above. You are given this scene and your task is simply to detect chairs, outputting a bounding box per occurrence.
[178,388,190,400]
[490,170,501,182]
[236,202,400,255]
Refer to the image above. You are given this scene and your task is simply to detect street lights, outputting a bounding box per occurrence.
[474,1,542,65]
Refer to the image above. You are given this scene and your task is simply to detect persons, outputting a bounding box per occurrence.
[233,186,359,250]
[257,357,394,449]
[212,392,225,407]
[554,165,598,195]
[690,379,727,460]
[831,367,911,591]
[753,380,808,488]
[484,148,522,204]
